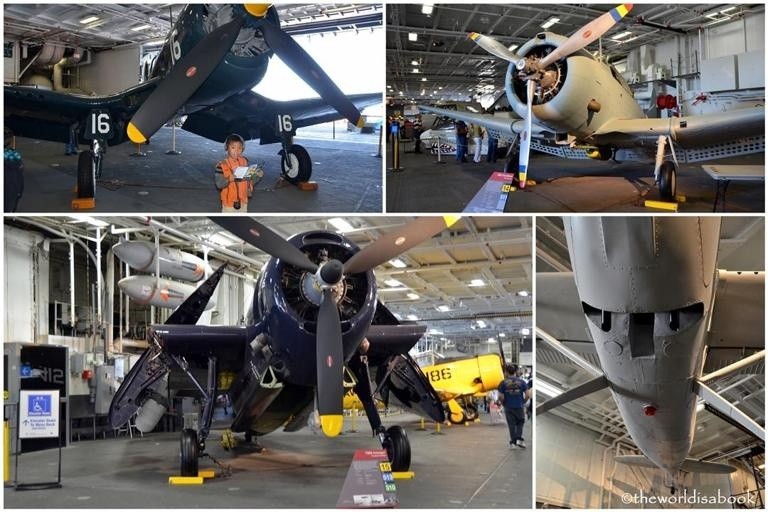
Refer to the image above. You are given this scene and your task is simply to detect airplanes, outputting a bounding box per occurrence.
[4,4,383,200]
[535,216,766,479]
[418,3,765,202]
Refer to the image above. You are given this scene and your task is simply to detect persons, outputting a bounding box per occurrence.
[486,128,501,163]
[470,123,484,163]
[496,365,529,450]
[213,134,264,213]
[387,113,423,154]
[455,119,468,163]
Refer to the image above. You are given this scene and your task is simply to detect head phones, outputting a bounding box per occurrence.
[223,133,245,152]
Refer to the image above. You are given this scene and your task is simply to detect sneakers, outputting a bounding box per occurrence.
[509,438,527,450]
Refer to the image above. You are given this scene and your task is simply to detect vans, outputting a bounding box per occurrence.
[428,102,482,115]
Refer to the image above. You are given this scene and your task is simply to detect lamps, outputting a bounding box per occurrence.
[325,216,355,233]
[130,23,151,32]
[80,14,101,24]
[387,256,409,269]
[408,5,563,100]
